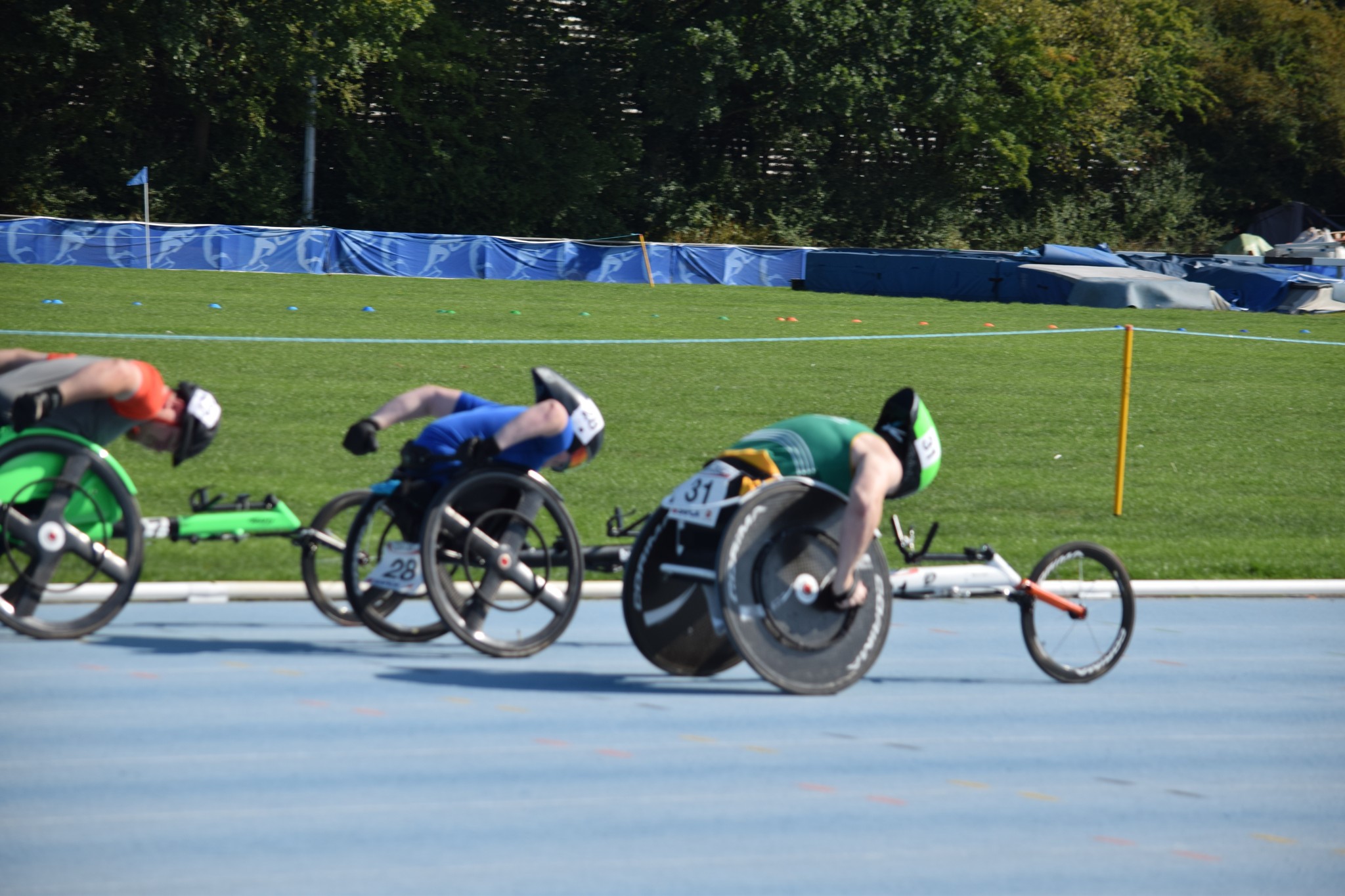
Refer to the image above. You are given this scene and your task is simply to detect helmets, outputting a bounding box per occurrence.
[531,367,605,472]
[171,379,221,467]
[873,385,941,499]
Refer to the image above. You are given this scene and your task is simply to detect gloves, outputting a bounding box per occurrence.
[12,386,63,432]
[341,418,382,455]
[455,436,502,462]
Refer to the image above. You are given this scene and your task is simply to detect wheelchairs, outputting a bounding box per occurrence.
[0,413,413,641]
[622,477,1138,701]
[292,435,633,660]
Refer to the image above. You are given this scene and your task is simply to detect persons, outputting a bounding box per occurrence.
[0,348,222,468]
[676,386,942,573]
[343,367,608,541]
